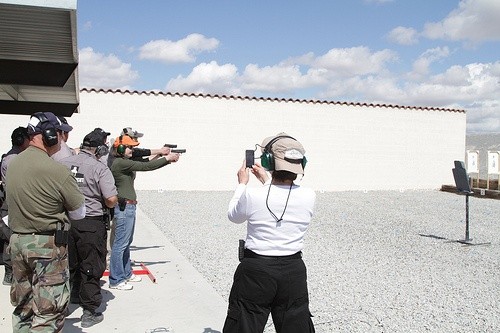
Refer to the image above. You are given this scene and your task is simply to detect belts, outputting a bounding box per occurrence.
[85,216,103,220]
[243,249,302,257]
[12,230,54,236]
[125,199,138,204]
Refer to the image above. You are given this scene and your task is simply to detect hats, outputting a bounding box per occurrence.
[261,132,306,174]
[28,112,73,135]
[113,135,140,148]
[83,131,104,147]
[93,128,110,138]
[120,127,143,138]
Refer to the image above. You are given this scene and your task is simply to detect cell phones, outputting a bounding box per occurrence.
[246,150,254,168]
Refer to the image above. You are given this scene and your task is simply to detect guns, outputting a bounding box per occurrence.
[171,148,186,154]
[159,143,177,157]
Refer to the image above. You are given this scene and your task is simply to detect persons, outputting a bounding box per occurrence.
[57,128,120,327]
[104,134,180,292]
[44,118,79,171]
[223,133,318,333]
[108,126,172,260]
[4,112,87,333]
[2,126,38,286]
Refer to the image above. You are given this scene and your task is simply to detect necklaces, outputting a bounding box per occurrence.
[265,176,293,223]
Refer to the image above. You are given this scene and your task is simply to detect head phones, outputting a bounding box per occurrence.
[260,135,307,170]
[80,128,128,158]
[14,111,58,147]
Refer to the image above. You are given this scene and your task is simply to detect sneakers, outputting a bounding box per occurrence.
[109,282,133,290]
[127,274,142,282]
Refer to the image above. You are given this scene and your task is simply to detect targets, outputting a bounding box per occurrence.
[451,160,471,193]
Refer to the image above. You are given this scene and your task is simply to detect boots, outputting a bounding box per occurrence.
[2,265,13,284]
[81,310,105,330]
